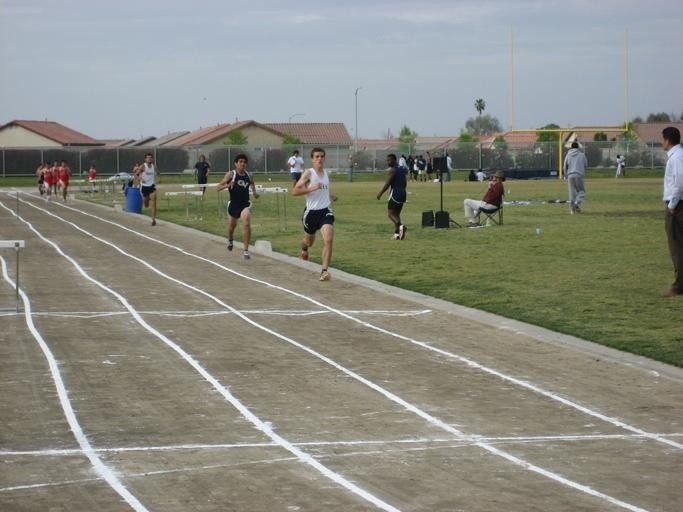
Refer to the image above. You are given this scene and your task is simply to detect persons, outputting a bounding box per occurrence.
[376,155,407,239]
[346,153,353,181]
[562,142,587,214]
[463,171,505,228]
[216,155,259,259]
[290,148,338,281]
[616,155,626,178]
[398,151,485,182]
[286,150,303,187]
[35,154,96,203]
[133,163,139,181]
[193,154,211,195]
[660,126,681,297]
[134,153,161,225]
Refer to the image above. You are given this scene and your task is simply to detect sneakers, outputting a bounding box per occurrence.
[227,239,233,250]
[391,233,400,239]
[244,249,249,259]
[319,271,328,281]
[301,247,309,260]
[399,225,407,240]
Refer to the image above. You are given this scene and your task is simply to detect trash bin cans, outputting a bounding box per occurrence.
[125,187,142,212]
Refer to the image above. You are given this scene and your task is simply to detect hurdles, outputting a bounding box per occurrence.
[0,240,24,314]
[165,183,288,233]
[0,189,23,220]
[44,177,135,201]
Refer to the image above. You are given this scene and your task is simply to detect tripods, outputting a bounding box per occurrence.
[434,174,461,229]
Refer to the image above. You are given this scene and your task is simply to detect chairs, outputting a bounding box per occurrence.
[473,192,504,226]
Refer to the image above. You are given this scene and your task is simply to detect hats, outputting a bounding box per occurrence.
[490,170,506,181]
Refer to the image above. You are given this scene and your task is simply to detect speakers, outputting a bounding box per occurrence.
[422,210,433,226]
[435,211,449,228]
[432,156,449,172]
